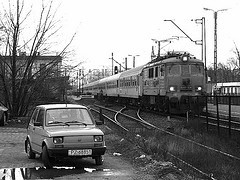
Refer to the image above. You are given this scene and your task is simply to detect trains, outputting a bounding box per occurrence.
[77,50,209,117]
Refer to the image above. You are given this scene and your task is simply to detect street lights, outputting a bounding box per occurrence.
[164,17,209,84]
[127,54,140,69]
[109,58,121,72]
[203,7,228,96]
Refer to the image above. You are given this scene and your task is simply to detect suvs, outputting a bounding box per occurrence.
[25,104,106,166]
